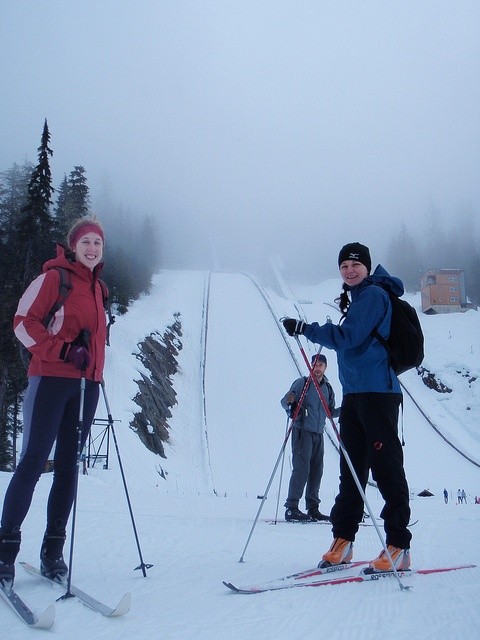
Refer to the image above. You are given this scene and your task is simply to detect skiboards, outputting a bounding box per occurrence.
[267,509,420,529]
[0,561,134,631]
[218,561,476,596]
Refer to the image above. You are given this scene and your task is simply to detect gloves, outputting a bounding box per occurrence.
[66,345,89,368]
[282,319,306,336]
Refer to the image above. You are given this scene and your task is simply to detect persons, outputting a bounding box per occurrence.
[0,214,109,581]
[282,241,413,574]
[280,354,340,521]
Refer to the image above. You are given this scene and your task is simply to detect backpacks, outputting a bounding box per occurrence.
[338,286,424,376]
[14,266,116,371]
[290,376,334,419]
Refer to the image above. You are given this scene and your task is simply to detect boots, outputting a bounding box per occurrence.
[0,530,22,587]
[40,535,68,577]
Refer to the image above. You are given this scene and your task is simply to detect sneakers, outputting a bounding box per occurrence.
[307,507,330,521]
[285,509,314,521]
[368,543,410,572]
[322,537,353,564]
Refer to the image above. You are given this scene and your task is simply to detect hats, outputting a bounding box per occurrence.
[338,243,372,271]
[311,354,327,364]
